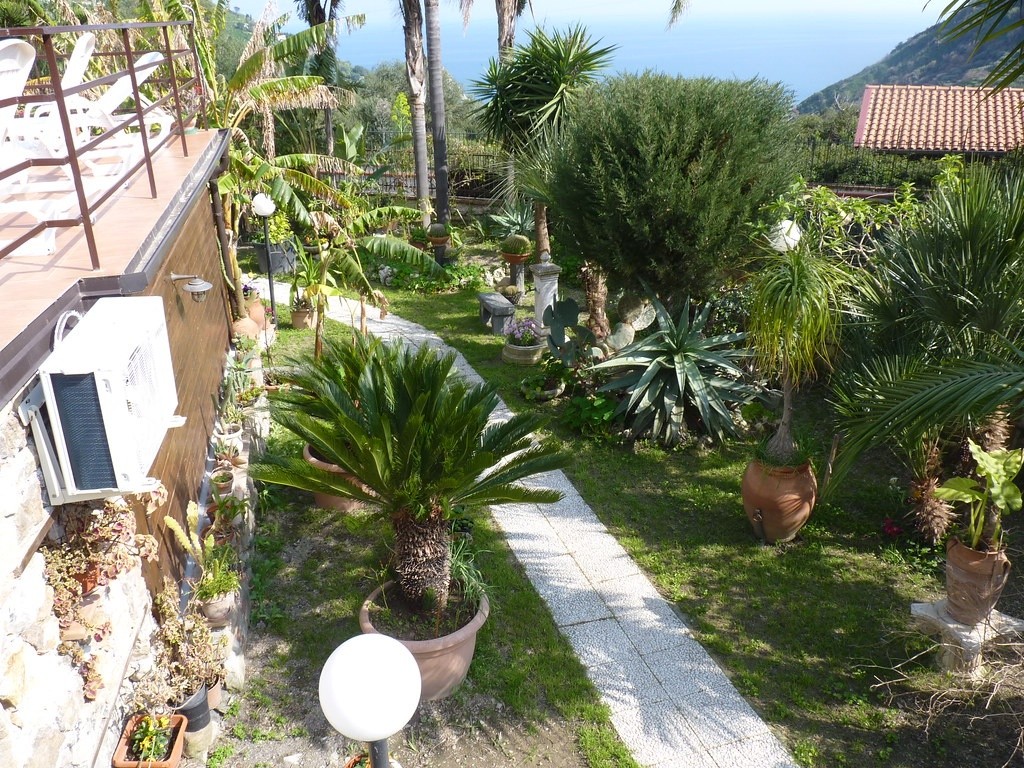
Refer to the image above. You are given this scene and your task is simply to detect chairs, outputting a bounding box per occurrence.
[0,32,175,257]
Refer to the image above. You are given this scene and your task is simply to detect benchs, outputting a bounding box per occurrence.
[477,292,517,335]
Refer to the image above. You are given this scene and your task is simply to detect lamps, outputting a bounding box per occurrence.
[252,193,277,216]
[318,633,422,742]
[769,219,802,253]
[170,271,213,302]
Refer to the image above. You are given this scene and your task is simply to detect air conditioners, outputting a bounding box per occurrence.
[38,296,178,496]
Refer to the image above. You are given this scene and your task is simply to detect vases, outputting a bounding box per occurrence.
[303,440,380,512]
[243,296,270,330]
[502,339,546,368]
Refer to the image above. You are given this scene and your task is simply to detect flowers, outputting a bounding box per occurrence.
[501,318,537,346]
[242,284,260,300]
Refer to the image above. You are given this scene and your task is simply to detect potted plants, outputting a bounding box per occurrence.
[426,223,449,246]
[155,87,197,132]
[251,209,295,276]
[247,209,329,328]
[518,351,578,402]
[40,483,169,701]
[501,285,523,305]
[501,235,532,265]
[242,325,588,702]
[729,211,861,545]
[408,225,428,252]
[930,438,1024,628]
[263,367,292,394]
[111,332,264,768]
